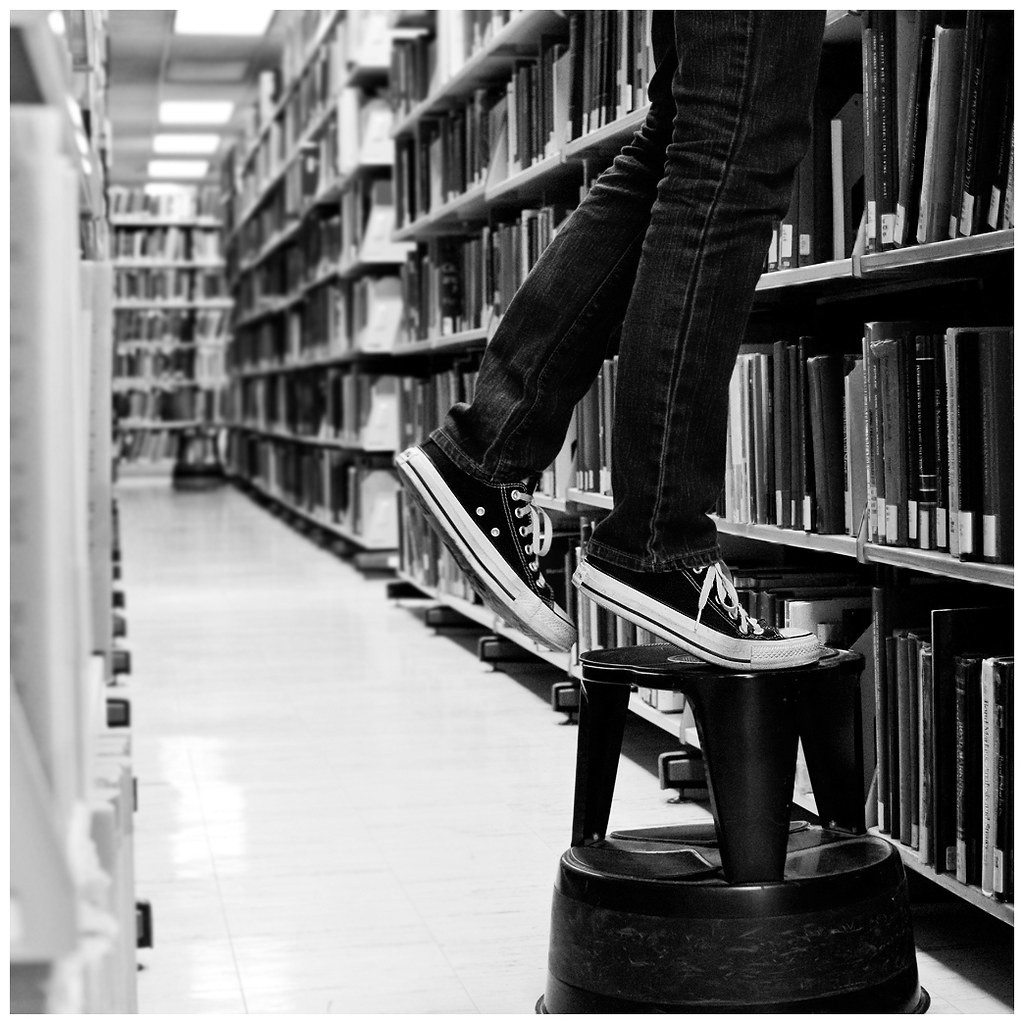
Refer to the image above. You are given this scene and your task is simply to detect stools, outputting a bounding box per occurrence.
[168,425,221,465]
[569,643,868,881]
[535,818,930,1014]
[172,461,227,491]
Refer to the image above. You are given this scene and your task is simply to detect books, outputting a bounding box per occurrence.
[8,9,1013,1015]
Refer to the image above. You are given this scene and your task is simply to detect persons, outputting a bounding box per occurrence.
[396,9,822,672]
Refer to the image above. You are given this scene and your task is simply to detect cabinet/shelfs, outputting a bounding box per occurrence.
[10,10,1014,1014]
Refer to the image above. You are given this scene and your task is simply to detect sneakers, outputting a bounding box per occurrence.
[396,439,579,653]
[572,554,822,670]
